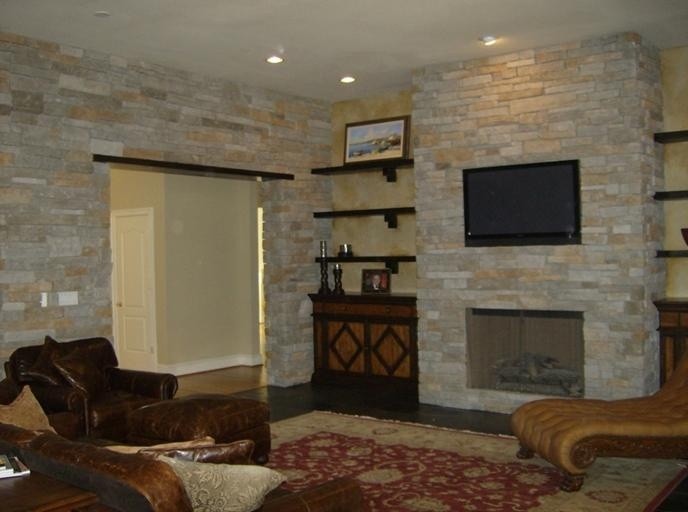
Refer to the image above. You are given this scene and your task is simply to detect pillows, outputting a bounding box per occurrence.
[136,440,255,465]
[102,436,215,454]
[156,455,286,512]
[0,384,57,434]
[53,346,118,402]
[24,334,70,388]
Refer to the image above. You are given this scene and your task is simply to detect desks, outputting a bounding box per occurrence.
[653,297,688,389]
[0,463,101,512]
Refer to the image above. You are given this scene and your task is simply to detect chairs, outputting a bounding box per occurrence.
[509,344,688,491]
[4,336,179,441]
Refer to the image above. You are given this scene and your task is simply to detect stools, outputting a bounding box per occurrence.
[126,394,271,464]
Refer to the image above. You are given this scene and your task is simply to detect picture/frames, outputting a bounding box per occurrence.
[344,114,411,166]
[360,269,390,293]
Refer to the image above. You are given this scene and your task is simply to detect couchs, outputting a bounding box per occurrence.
[0,379,368,512]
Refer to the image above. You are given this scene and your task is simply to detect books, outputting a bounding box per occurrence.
[1,453,32,480]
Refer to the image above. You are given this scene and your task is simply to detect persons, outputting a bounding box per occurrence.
[364,273,388,294]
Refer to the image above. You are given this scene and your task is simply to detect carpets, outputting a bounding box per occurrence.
[264,410,688,512]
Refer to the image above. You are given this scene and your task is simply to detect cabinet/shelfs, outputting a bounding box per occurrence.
[311,158,416,274]
[653,129,688,257]
[306,292,417,392]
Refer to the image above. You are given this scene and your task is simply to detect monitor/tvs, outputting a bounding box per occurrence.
[460,160,580,247]
